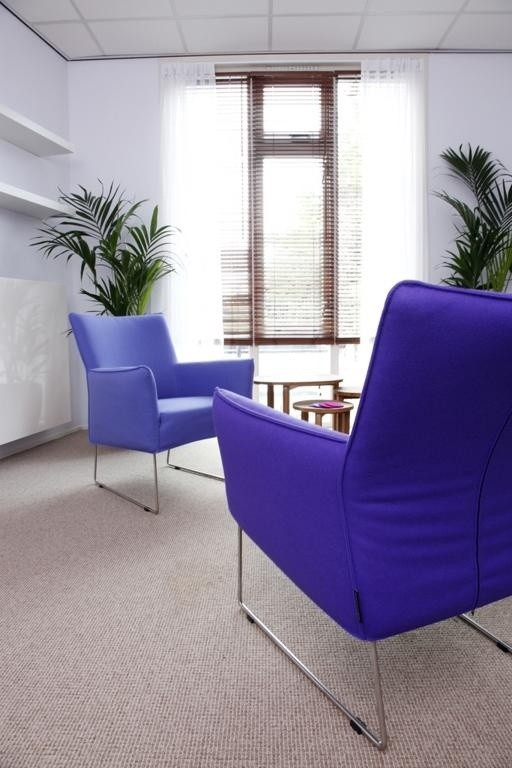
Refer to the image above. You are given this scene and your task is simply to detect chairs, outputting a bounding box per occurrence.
[210,278,512,752]
[68,310,255,516]
